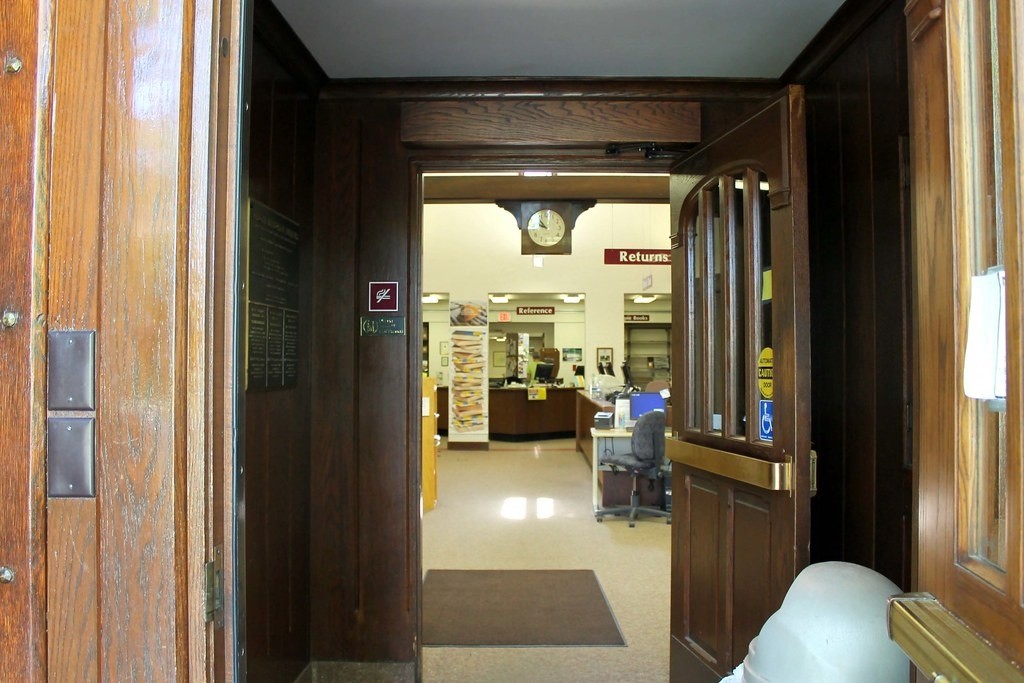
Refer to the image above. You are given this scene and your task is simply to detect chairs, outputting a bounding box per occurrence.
[646,380,669,393]
[594,411,672,528]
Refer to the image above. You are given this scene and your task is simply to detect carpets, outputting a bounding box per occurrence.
[422,569,628,648]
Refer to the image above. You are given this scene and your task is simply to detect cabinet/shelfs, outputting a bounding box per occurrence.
[422,376,437,513]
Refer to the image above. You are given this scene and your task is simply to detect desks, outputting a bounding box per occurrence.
[574,389,672,492]
[590,427,672,511]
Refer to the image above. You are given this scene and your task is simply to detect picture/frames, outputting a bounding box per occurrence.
[597,348,613,367]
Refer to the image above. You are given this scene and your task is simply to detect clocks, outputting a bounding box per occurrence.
[495,198,597,254]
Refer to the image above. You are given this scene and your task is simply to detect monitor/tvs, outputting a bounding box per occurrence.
[535,364,554,382]
[630,392,667,421]
[598,361,636,392]
[574,366,585,377]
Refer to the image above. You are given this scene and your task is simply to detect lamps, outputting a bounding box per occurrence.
[564,294,580,303]
[421,293,438,303]
[491,293,508,304]
[962,265,1007,411]
[633,295,657,304]
